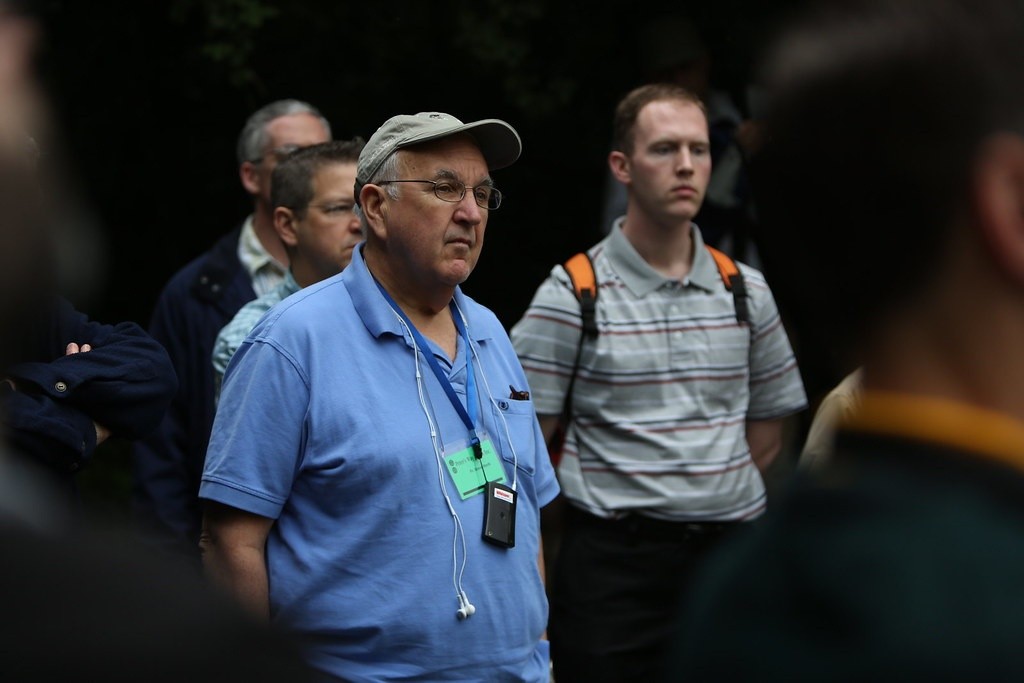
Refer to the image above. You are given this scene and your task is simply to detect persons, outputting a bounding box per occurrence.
[0,1,1024,682]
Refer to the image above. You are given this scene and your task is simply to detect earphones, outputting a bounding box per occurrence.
[457,594,466,619]
[461,591,475,615]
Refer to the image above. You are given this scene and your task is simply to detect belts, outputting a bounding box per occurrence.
[581,513,740,547]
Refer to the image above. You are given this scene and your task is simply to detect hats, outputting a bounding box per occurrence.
[355,112,522,185]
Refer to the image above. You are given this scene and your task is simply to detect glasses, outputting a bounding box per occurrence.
[251,146,304,163]
[376,179,503,210]
